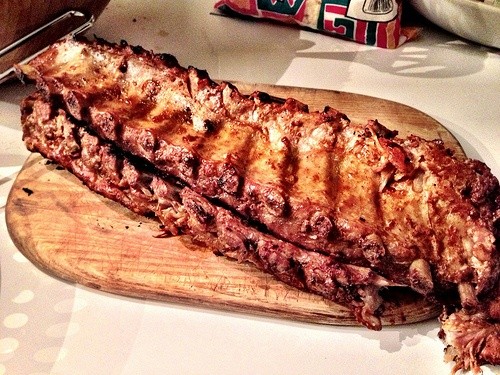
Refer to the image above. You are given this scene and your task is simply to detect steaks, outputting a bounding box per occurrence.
[13,32,500,309]
[20,89,435,331]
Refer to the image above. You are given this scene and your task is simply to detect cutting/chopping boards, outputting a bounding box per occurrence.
[4,79,470,327]
